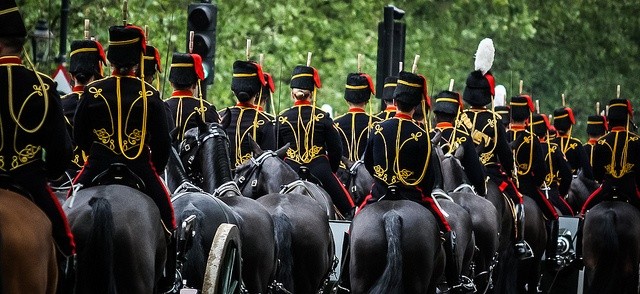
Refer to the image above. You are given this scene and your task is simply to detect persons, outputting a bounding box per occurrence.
[135,44,176,137]
[254,72,276,113]
[0,0,80,293]
[582,114,610,180]
[162,51,230,188]
[493,106,511,129]
[428,89,489,197]
[331,72,385,163]
[573,99,640,270]
[526,113,578,218]
[217,60,278,179]
[336,70,464,294]
[48,39,107,193]
[72,24,177,293]
[276,65,355,221]
[505,96,566,271]
[460,69,533,260]
[546,125,558,141]
[553,106,584,180]
[373,74,400,121]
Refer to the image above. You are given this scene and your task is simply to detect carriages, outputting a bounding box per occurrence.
[169,107,340,294]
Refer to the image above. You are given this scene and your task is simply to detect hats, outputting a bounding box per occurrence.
[494,84,510,124]
[107,0,146,63]
[463,38,495,107]
[433,79,464,114]
[169,31,204,84]
[0,0,27,37]
[145,25,161,75]
[231,39,266,91]
[69,19,107,72]
[382,61,403,99]
[510,80,535,114]
[393,55,427,106]
[345,53,375,103]
[290,52,321,90]
[554,94,575,125]
[257,53,274,99]
[532,100,551,130]
[608,85,633,120]
[587,101,608,134]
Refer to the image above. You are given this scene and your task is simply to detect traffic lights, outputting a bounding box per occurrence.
[186,3,218,84]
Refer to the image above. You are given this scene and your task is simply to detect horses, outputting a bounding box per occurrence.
[62,184,167,293]
[437,141,498,294]
[0,188,58,293]
[431,129,472,293]
[568,168,640,294]
[178,107,274,293]
[233,132,334,293]
[161,125,238,294]
[338,156,446,294]
[476,137,513,293]
[507,138,548,293]
